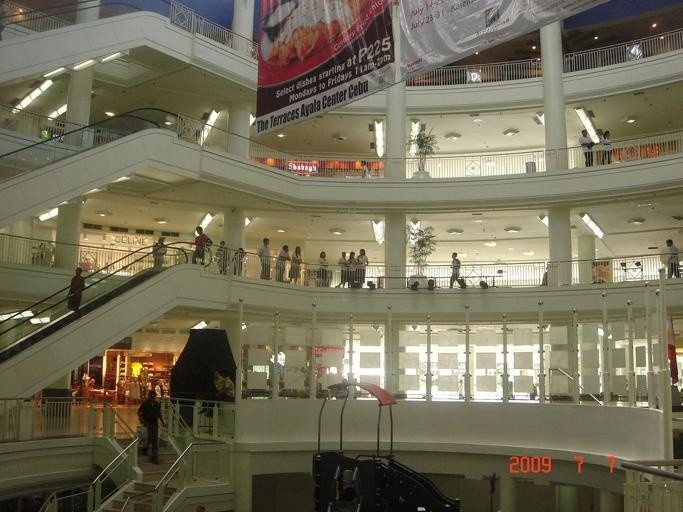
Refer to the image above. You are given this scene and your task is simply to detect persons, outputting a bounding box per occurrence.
[216,240,228,275]
[66,267,85,316]
[80,374,168,405]
[257,238,370,289]
[152,236,167,269]
[360,159,369,179]
[578,129,595,166]
[136,389,167,464]
[449,252,461,288]
[192,226,213,266]
[601,130,613,165]
[232,247,247,276]
[665,239,680,278]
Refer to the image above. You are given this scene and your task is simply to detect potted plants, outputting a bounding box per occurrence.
[403,121,443,180]
[400,226,437,288]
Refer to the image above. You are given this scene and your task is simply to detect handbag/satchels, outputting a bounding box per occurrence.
[136,426,149,449]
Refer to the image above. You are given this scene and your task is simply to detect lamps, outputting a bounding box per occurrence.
[188,319,211,331]
[11,77,52,116]
[573,104,601,147]
[194,210,214,240]
[370,216,385,247]
[370,117,386,158]
[406,218,421,244]
[194,108,221,147]
[1,308,36,323]
[405,118,420,153]
[578,209,605,239]
[535,109,546,125]
[534,214,551,229]
